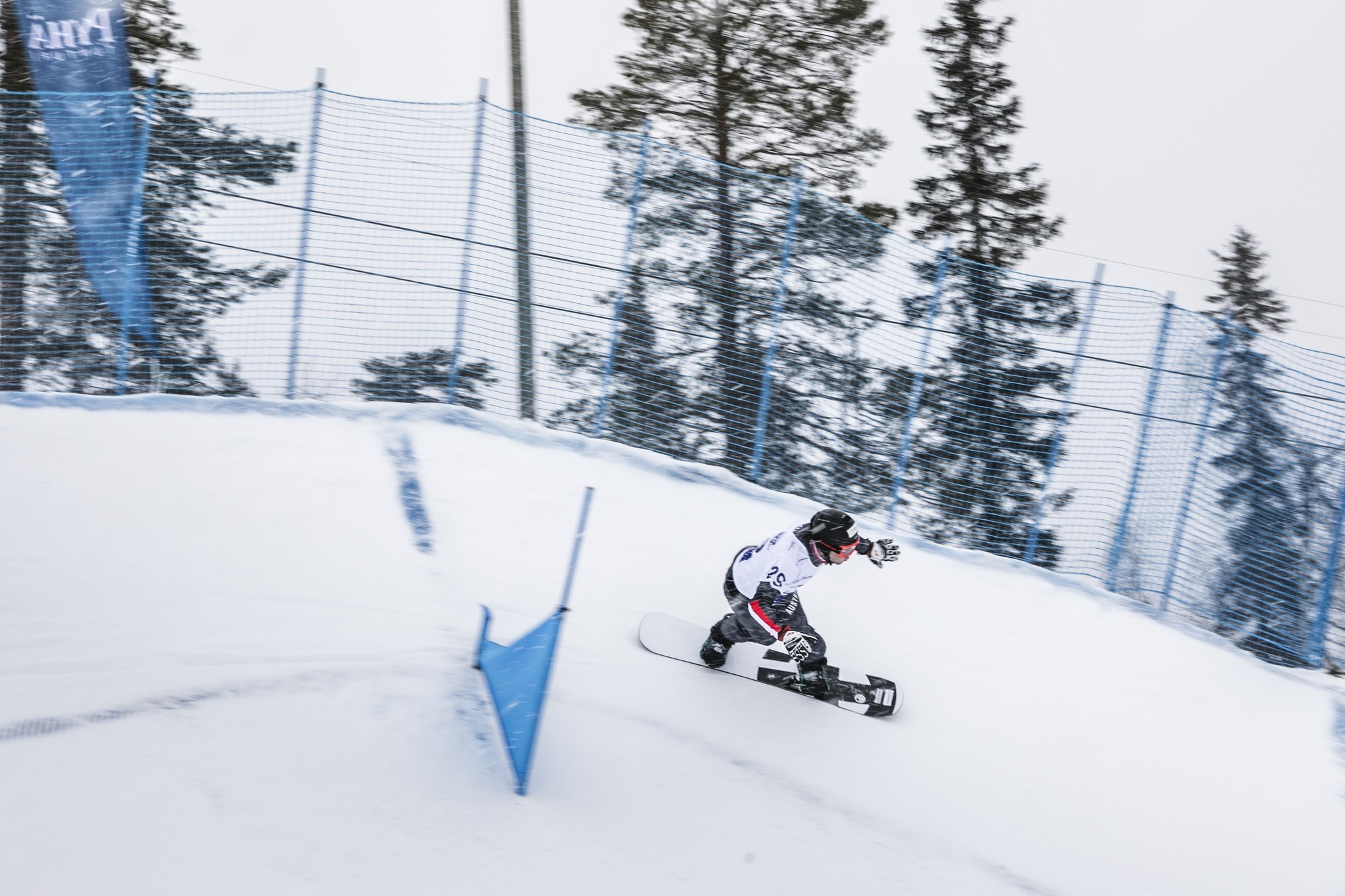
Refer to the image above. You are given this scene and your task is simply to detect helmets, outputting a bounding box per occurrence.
[810,509,857,554]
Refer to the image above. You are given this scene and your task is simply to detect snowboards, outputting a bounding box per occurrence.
[638,611,904,717]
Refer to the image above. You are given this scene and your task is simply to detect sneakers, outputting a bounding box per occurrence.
[700,613,735,668]
[798,656,828,693]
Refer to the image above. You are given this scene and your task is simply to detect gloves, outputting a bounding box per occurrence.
[867,539,900,568]
[783,630,818,663]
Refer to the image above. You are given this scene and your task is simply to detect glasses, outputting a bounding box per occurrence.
[840,537,860,557]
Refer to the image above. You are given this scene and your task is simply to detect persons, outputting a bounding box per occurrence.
[699,508,901,699]
